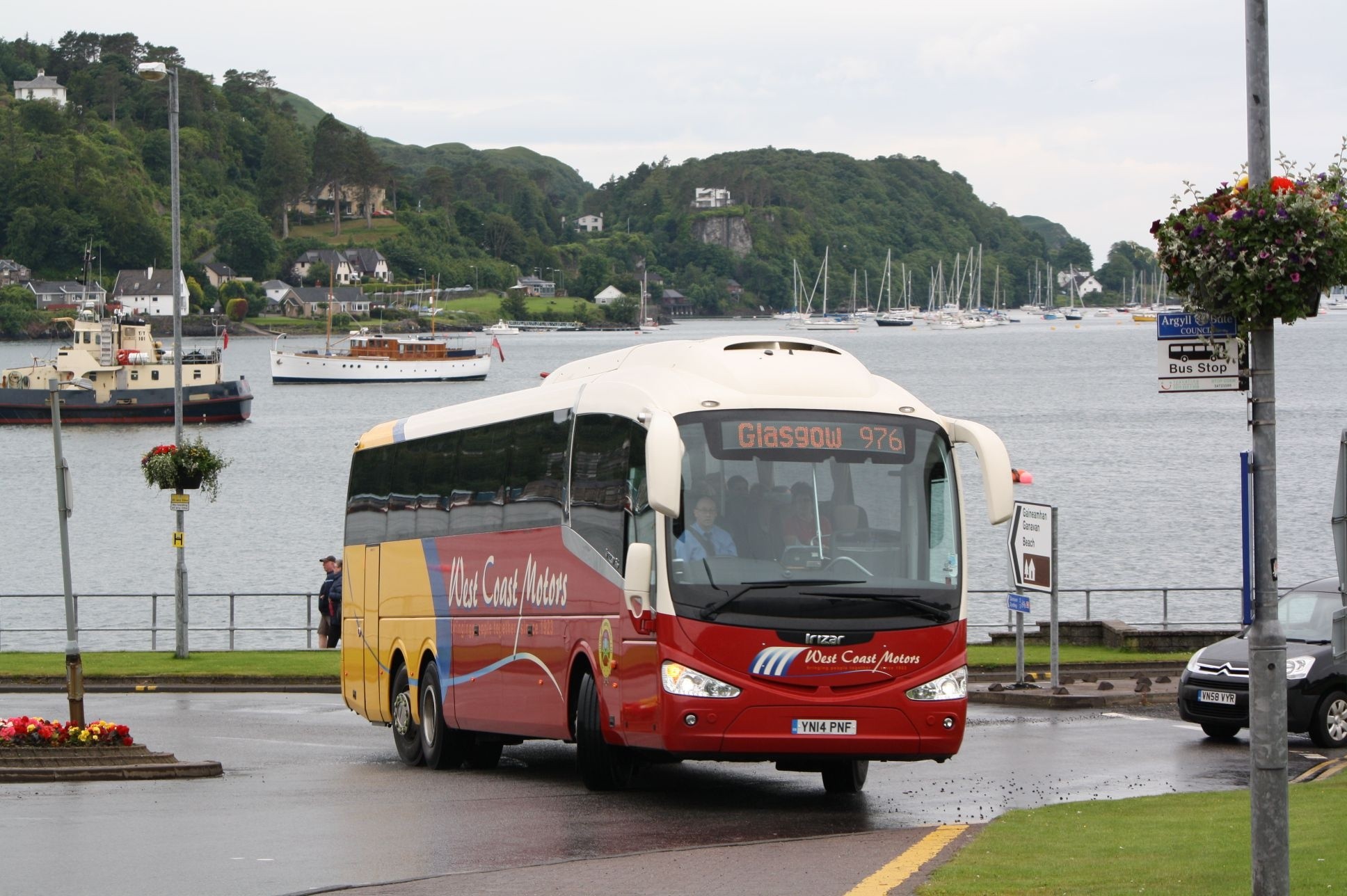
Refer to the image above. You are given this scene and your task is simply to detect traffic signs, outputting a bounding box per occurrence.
[1006,501,1054,595]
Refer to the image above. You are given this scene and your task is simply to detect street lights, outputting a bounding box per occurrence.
[419,268,426,292]
[470,265,478,291]
[534,267,565,289]
[222,266,231,282]
[510,265,518,284]
[135,60,189,663]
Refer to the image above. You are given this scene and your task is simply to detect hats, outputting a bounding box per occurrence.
[319,556,335,562]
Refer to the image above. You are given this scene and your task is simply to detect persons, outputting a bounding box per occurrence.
[317,556,345,648]
[676,474,835,561]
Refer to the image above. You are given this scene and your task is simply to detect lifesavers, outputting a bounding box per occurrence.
[116,349,126,362]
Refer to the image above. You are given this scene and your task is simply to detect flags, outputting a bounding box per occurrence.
[492,334,504,362]
[223,330,228,350]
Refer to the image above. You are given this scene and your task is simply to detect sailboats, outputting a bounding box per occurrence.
[638,270,661,334]
[771,240,1089,331]
[1114,267,1182,322]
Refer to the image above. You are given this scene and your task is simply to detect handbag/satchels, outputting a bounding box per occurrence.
[334,603,341,622]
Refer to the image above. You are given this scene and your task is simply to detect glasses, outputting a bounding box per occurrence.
[696,508,718,514]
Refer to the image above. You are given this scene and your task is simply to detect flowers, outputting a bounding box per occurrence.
[141,422,234,503]
[1150,134,1347,363]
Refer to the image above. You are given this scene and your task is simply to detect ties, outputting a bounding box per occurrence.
[703,530,715,554]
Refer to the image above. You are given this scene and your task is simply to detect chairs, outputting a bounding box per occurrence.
[830,504,869,544]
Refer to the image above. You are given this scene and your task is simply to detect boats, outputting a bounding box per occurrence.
[1095,309,1114,317]
[484,318,520,336]
[266,258,495,385]
[0,234,255,427]
[1317,286,1347,314]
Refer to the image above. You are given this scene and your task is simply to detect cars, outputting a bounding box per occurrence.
[1173,576,1347,753]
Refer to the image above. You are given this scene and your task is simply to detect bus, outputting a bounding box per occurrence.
[336,330,1019,798]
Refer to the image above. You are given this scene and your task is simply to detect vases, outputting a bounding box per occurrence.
[158,465,201,489]
